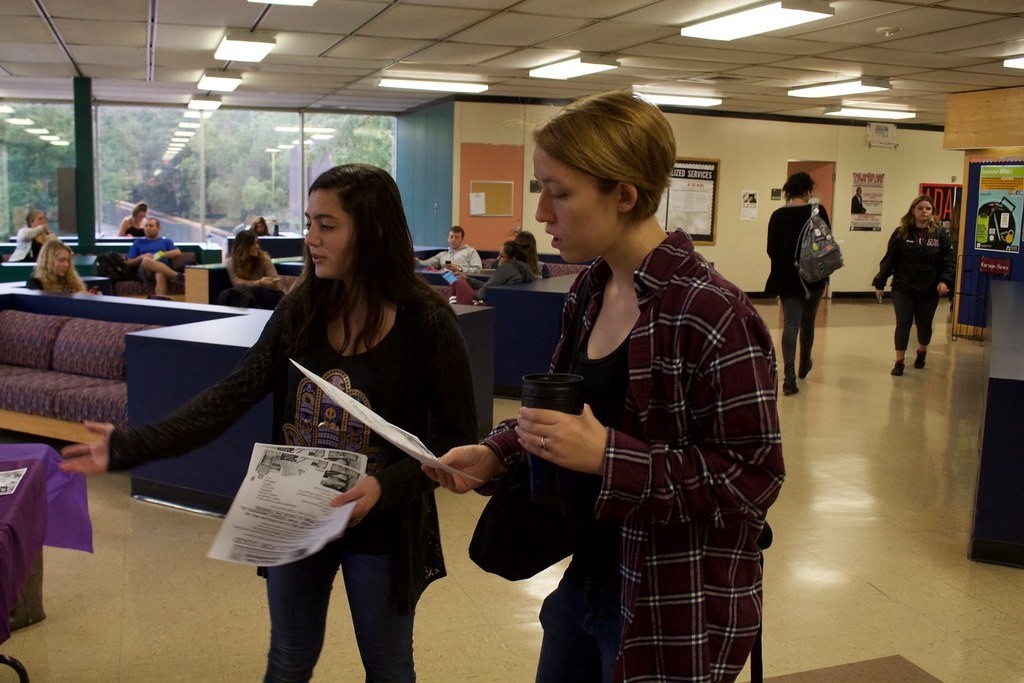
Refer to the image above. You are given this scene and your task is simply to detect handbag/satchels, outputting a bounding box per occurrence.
[469,449,573,582]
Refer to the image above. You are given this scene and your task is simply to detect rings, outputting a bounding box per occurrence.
[539,436,545,449]
[459,268,461,271]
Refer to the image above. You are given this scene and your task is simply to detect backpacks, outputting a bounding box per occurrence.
[217,284,257,308]
[794,204,844,284]
[96,251,136,282]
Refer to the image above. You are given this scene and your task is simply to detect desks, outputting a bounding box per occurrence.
[0,442,99,647]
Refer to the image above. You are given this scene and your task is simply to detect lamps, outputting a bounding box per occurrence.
[188,1,1024,121]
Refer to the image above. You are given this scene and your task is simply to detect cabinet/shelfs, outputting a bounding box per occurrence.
[968,279,1024,573]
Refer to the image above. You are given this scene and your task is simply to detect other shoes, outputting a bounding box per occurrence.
[798,358,812,379]
[782,382,799,394]
[891,358,905,376]
[914,347,927,369]
[176,272,185,282]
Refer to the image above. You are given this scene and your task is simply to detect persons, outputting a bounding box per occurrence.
[852,188,866,214]
[872,194,957,375]
[515,231,538,273]
[448,241,535,306]
[60,162,479,683]
[225,230,280,289]
[125,217,185,295]
[27,238,102,295]
[250,216,279,236]
[767,172,832,392]
[8,210,55,262]
[119,203,150,237]
[415,226,482,274]
[423,91,788,683]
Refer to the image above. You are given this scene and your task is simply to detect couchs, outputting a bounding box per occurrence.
[1,222,631,522]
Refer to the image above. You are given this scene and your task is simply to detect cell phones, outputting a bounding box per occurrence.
[445,260,452,265]
[440,271,458,285]
[271,278,282,282]
[89,285,98,294]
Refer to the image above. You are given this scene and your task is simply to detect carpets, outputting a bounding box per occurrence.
[738,651,947,683]
